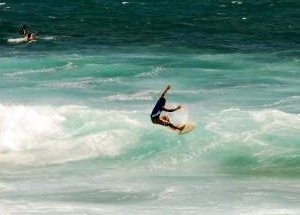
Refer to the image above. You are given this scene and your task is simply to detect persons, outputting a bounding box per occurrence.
[20,23,40,42]
[150,85,185,132]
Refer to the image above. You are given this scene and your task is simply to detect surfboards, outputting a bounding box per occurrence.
[173,124,195,133]
